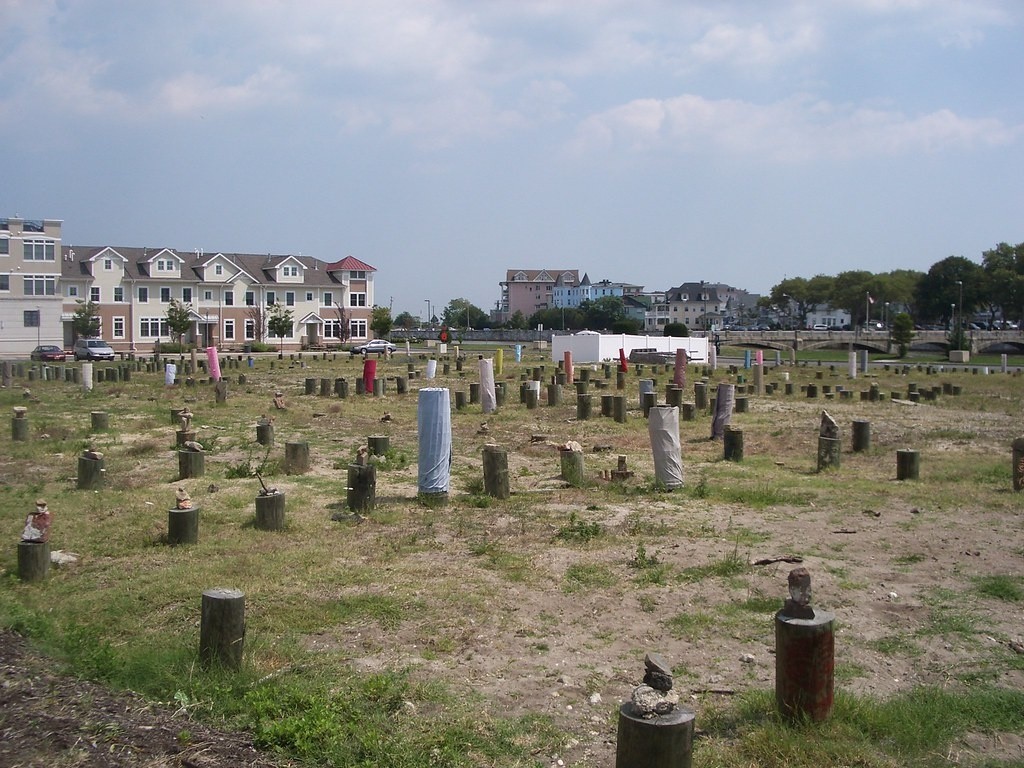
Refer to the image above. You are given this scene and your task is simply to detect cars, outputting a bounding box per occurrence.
[31,345,66,363]
[350,338,397,356]
[812,319,1021,332]
[724,322,771,331]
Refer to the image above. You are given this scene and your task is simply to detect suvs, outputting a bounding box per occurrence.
[73,338,116,362]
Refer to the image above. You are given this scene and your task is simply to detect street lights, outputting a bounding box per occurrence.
[955,281,963,350]
[425,299,430,323]
[885,301,891,354]
[951,303,956,351]
